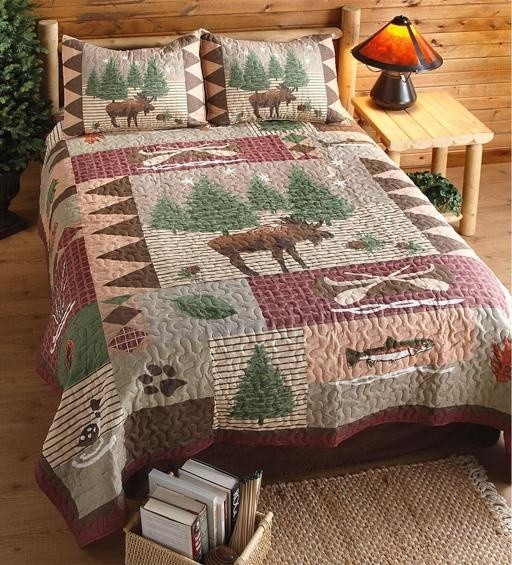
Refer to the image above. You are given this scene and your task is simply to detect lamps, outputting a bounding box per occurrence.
[348,15,447,109]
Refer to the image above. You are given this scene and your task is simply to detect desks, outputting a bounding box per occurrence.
[351,93,494,239]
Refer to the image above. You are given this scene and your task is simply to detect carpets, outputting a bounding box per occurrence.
[248,448,512,565]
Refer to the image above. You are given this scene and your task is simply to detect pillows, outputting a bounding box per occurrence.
[56,28,211,139]
[196,26,348,129]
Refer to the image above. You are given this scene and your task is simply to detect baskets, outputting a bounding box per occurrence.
[123,506,272,565]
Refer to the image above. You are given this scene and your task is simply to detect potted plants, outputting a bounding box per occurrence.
[0,0,62,239]
[409,171,463,232]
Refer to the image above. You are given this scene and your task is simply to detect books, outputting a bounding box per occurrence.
[139,455,241,562]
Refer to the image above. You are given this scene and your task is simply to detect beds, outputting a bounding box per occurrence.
[35,3,509,550]
